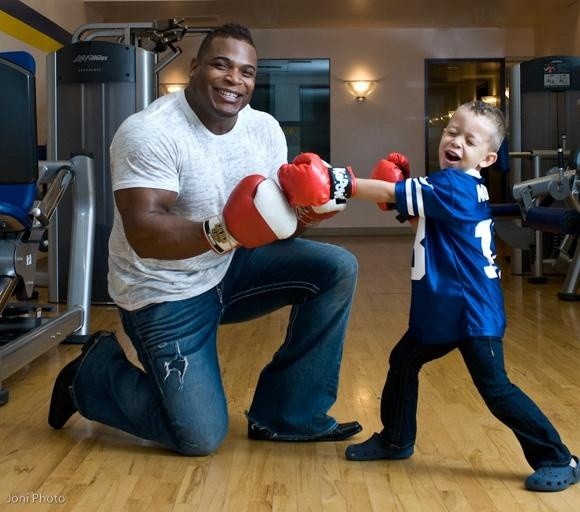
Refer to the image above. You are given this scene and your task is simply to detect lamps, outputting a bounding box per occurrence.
[343,79,378,102]
[448,110,455,118]
[481,96,497,103]
[159,82,189,96]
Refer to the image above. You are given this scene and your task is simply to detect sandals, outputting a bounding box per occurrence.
[525,454,580,492]
[346,431,414,461]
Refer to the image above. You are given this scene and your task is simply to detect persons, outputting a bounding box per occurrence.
[47,22,364,457]
[277,100,580,491]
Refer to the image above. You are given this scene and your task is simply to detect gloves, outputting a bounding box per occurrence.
[279,153,356,205]
[371,153,419,224]
[203,175,298,255]
[294,158,347,237]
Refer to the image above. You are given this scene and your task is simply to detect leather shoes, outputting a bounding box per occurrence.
[48,330,107,429]
[247,421,362,442]
